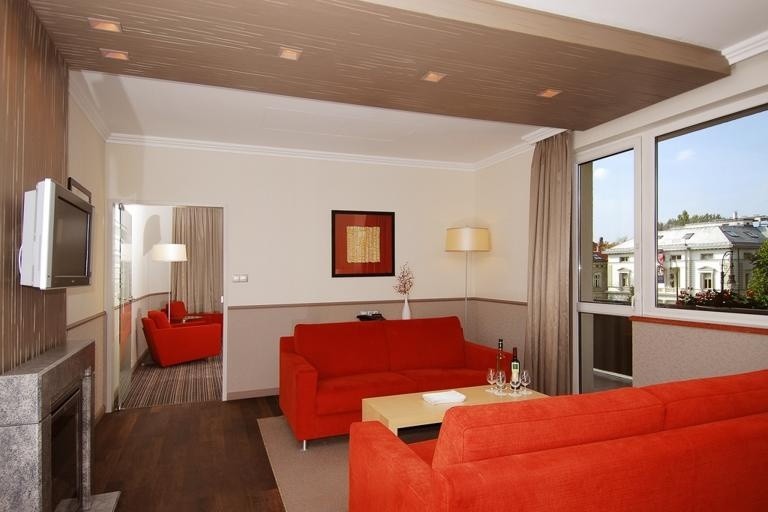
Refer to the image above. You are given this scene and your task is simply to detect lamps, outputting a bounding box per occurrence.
[445,226,492,341]
[150,243,188,326]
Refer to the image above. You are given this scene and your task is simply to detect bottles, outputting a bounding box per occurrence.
[495,339,507,389]
[509,347,520,390]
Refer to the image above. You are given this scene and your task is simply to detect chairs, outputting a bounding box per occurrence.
[142,299,222,367]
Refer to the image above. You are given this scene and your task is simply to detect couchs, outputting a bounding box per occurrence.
[279,315,518,452]
[348,367,768,511]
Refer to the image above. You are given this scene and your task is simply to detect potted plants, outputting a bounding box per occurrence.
[391,263,416,320]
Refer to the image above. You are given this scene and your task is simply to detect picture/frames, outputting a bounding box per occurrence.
[331,210,396,278]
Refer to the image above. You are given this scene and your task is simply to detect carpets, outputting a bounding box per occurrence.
[256,415,349,512]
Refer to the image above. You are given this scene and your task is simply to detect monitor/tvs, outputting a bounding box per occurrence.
[18,178,94,291]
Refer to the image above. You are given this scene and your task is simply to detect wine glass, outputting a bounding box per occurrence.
[486,368,497,393]
[520,370,533,395]
[495,371,507,396]
[510,371,522,398]
